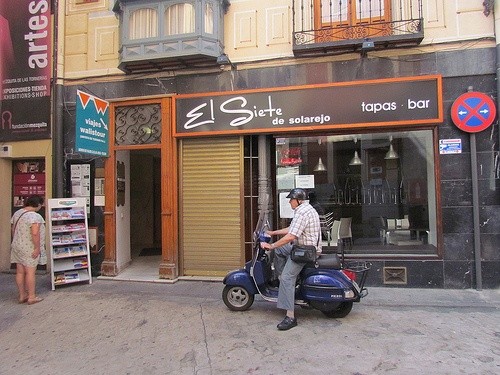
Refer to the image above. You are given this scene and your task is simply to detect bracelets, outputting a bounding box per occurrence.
[271,243,276,250]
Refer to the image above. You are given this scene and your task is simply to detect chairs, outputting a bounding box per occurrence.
[322,218,353,249]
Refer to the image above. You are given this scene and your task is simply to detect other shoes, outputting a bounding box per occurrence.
[28,297,43,304]
[268,277,280,287]
[18,297,28,303]
[277,315,298,331]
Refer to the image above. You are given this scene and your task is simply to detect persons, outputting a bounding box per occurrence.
[16,196,23,205]
[8,195,48,306]
[261,188,323,329]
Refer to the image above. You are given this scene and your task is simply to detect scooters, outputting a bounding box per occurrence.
[222,209,371,318]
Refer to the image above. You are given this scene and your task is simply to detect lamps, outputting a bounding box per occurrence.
[384,136,400,159]
[348,138,364,165]
[313,139,327,171]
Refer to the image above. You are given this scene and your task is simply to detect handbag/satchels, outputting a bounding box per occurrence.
[291,243,316,263]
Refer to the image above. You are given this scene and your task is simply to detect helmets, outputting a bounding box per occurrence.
[286,188,310,200]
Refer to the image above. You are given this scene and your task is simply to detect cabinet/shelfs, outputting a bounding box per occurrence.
[47,198,92,290]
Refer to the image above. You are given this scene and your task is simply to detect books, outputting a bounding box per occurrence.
[73,257,88,268]
[51,209,85,218]
[52,223,86,230]
[52,231,87,243]
[55,270,80,285]
[53,245,87,256]
